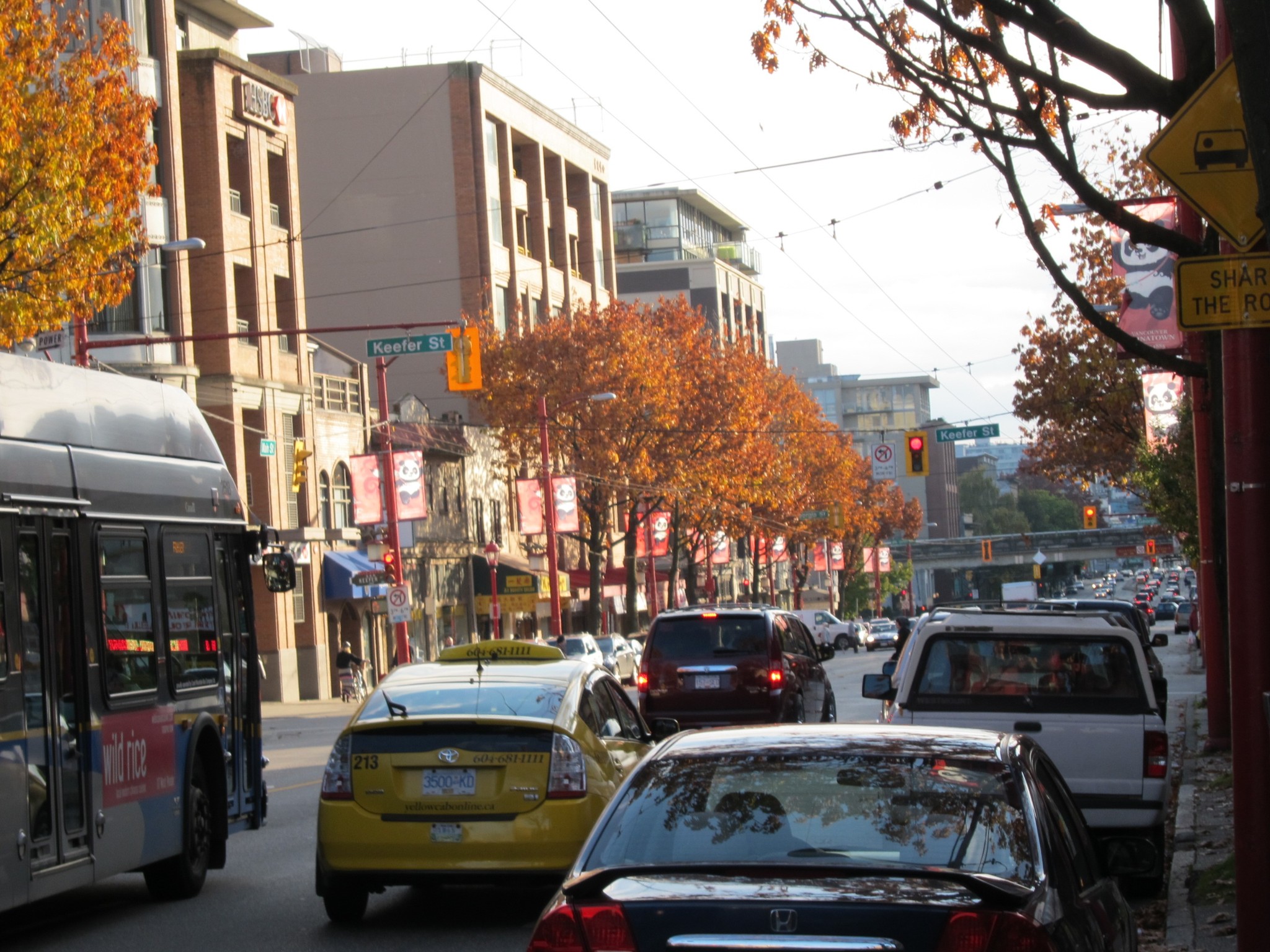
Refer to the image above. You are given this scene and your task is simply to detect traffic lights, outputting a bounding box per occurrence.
[1150,556,1157,568]
[384,552,395,584]
[902,590,906,601]
[1083,505,1096,529]
[904,431,928,477]
[969,593,973,597]
[744,580,750,595]
[982,540,992,561]
[291,440,313,493]
[1146,539,1155,555]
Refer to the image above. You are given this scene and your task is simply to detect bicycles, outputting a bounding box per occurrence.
[351,659,371,705]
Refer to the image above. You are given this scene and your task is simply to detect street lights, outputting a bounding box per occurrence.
[908,522,938,617]
[483,538,500,640]
[538,390,615,635]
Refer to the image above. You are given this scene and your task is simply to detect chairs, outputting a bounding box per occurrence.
[708,789,793,854]
[895,810,990,865]
[1041,651,1107,694]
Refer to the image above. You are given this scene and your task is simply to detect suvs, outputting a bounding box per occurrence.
[544,631,643,688]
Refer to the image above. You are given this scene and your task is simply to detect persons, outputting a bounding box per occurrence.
[849,618,859,652]
[1189,606,1201,648]
[821,622,834,660]
[446,636,455,647]
[336,642,370,702]
[889,617,911,661]
[557,636,568,658]
[391,635,415,667]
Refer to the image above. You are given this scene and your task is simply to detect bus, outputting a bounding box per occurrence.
[0,352,298,922]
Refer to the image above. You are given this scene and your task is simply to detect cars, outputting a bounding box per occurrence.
[788,549,1200,673]
[523,720,1158,952]
[315,641,681,923]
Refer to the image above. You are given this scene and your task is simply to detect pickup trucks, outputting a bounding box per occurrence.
[861,606,1172,900]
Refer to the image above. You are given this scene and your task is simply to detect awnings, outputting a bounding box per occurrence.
[324,550,387,600]
[472,553,571,598]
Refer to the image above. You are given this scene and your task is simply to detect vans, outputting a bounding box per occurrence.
[638,602,838,741]
